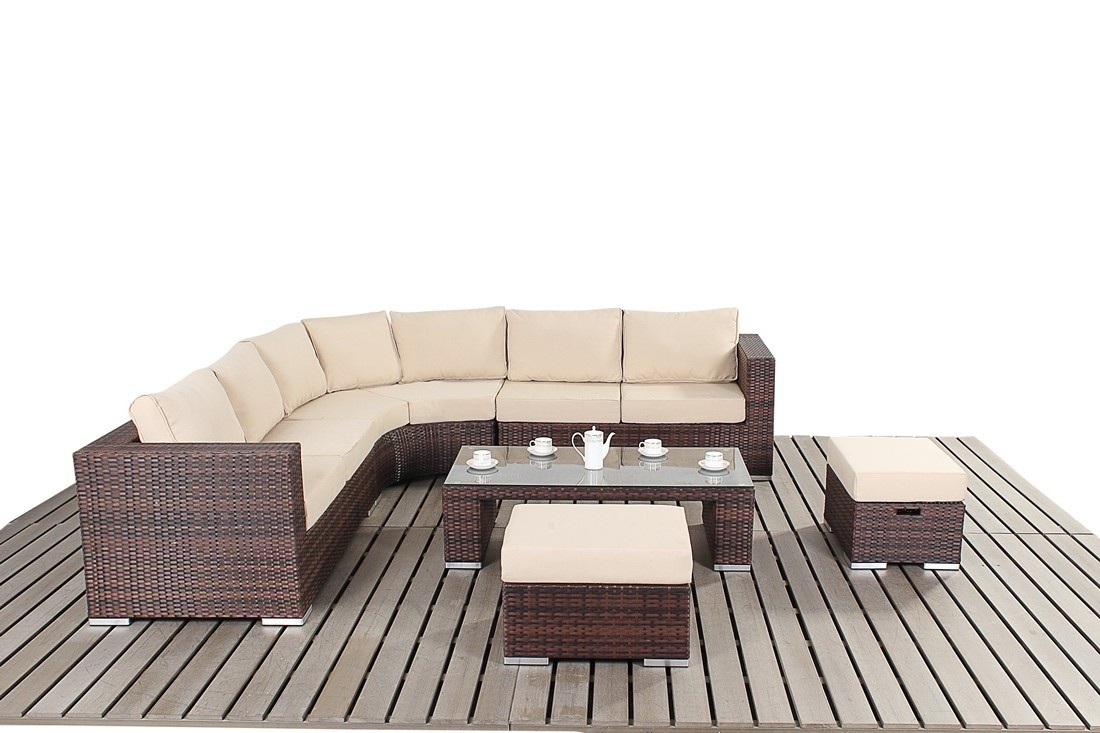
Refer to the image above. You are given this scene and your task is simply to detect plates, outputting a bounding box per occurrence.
[698,468,730,476]
[638,447,669,457]
[698,459,730,470]
[464,459,498,469]
[527,454,557,460]
[638,455,668,462]
[526,446,557,456]
[466,467,499,474]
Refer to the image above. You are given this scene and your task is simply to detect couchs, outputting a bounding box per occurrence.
[69,297,776,625]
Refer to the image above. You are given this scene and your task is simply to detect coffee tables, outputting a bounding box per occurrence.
[443,445,755,570]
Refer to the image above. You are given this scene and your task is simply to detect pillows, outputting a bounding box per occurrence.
[209,340,285,444]
[302,311,402,392]
[502,308,623,382]
[623,310,738,383]
[389,306,510,381]
[248,320,327,412]
[131,367,246,443]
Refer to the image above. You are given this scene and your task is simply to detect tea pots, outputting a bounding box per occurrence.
[572,425,616,470]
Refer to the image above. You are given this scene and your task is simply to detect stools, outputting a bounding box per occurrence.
[500,502,694,667]
[825,434,967,568]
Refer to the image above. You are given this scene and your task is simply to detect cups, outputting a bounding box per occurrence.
[529,437,553,453]
[529,459,553,471]
[639,438,663,455]
[473,474,491,483]
[705,476,723,486]
[473,449,491,467]
[638,460,662,471]
[705,451,723,468]
